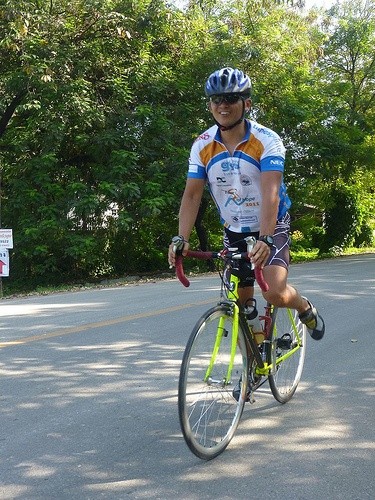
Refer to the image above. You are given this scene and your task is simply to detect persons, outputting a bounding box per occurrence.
[168,67,325,402]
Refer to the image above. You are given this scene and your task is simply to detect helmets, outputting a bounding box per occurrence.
[204,67,252,98]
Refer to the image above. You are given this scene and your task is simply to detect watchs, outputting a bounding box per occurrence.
[259,234,274,248]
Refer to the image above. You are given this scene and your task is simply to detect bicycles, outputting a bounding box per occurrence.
[169,236,307,460]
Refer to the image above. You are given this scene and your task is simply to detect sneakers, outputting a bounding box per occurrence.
[299,295,325,340]
[234,370,254,402]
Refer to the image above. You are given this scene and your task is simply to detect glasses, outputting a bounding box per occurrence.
[209,94,243,104]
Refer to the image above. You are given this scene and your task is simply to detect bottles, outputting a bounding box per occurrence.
[244,303,265,344]
